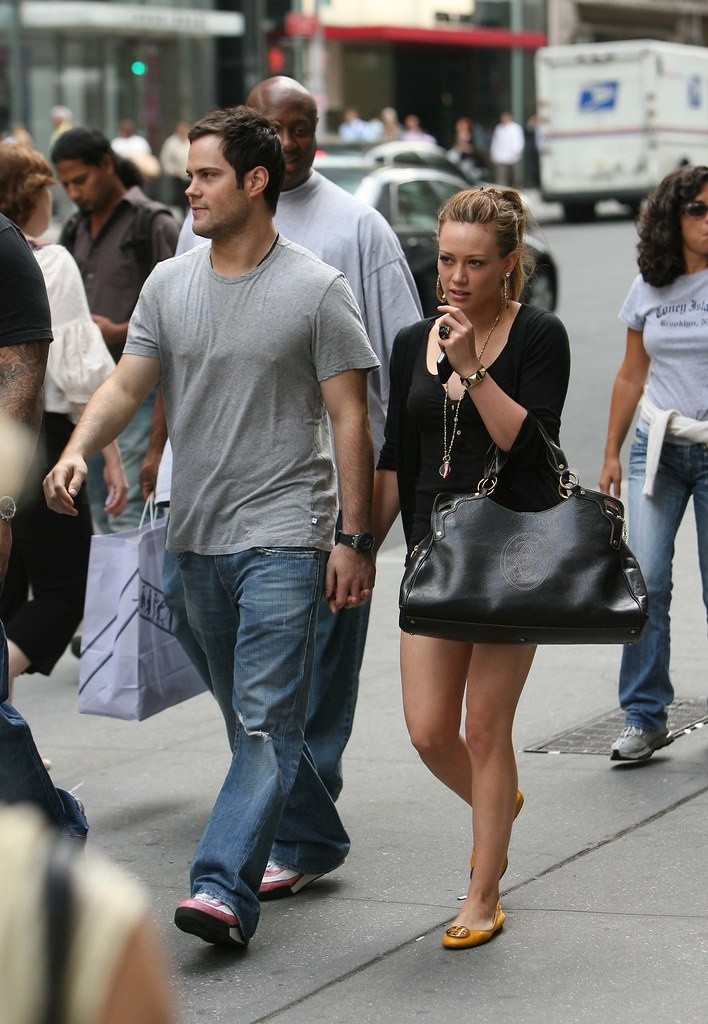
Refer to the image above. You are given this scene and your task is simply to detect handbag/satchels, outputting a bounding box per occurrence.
[79,492,210,723]
[399,422,648,644]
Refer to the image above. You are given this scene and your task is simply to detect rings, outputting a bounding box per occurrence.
[439,325,451,339]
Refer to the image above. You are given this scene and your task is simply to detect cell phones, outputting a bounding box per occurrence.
[436,352,454,385]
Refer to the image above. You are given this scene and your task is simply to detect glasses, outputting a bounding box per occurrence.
[679,201,708,218]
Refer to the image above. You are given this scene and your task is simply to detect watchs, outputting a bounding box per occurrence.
[0,495,17,522]
[335,530,375,555]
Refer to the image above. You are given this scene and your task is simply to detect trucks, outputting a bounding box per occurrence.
[532,36,708,224]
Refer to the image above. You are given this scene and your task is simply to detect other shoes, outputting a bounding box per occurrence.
[443,900,505,947]
[470,789,524,881]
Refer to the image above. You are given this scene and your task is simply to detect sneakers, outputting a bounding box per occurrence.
[257,861,325,901]
[611,720,674,761]
[175,892,247,949]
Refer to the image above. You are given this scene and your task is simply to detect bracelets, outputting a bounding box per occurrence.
[459,365,486,389]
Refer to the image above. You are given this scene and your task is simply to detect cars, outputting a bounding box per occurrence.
[315,140,558,328]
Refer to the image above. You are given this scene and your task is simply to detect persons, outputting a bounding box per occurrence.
[328,187,572,950]
[51,126,182,363]
[0,120,131,686]
[337,106,542,188]
[599,167,708,760]
[175,74,424,803]
[0,213,90,868]
[110,117,193,216]
[41,105,382,955]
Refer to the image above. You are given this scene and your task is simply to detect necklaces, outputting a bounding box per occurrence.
[438,294,506,479]
